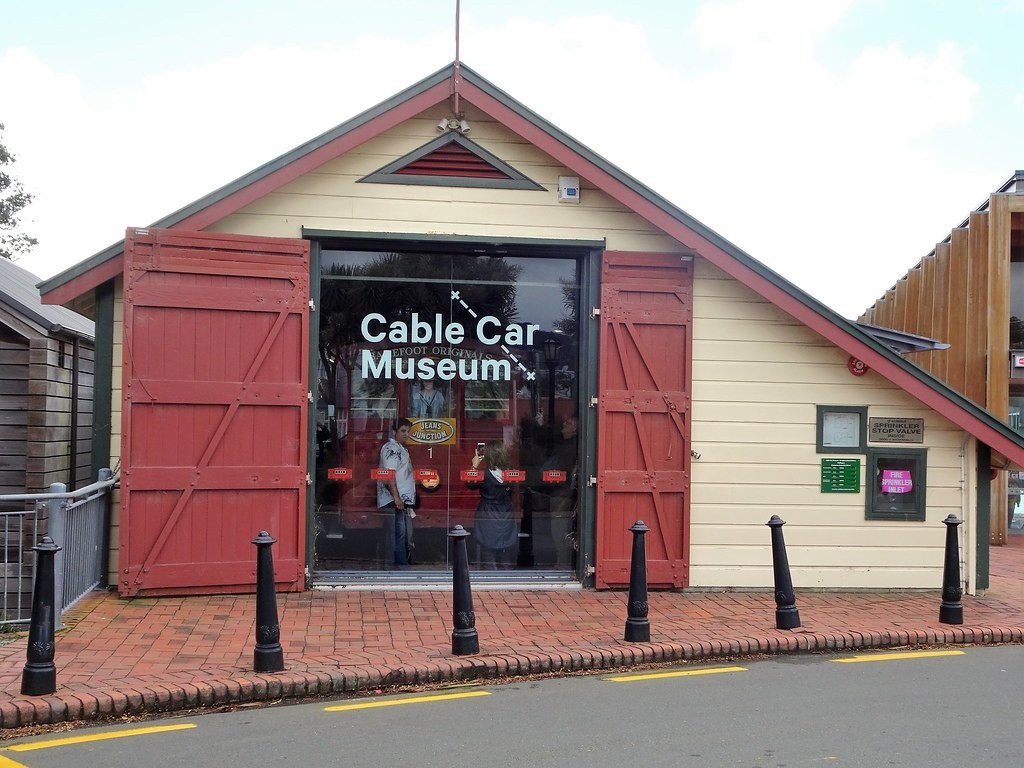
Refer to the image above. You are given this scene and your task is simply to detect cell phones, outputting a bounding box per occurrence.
[477,443,485,459]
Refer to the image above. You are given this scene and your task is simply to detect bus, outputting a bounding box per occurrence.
[330,335,524,533]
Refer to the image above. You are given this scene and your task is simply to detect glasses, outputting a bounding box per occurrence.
[563,420,574,426]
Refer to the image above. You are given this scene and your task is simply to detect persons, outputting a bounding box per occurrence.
[369,419,421,572]
[536,406,579,571]
[469,442,522,572]
[408,368,446,420]
